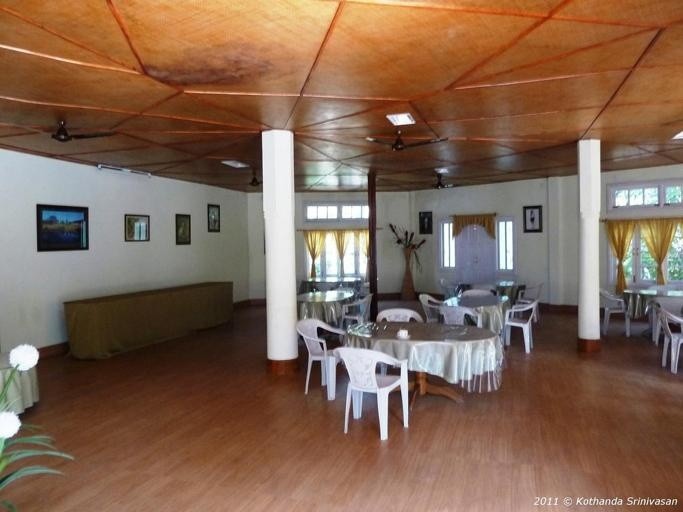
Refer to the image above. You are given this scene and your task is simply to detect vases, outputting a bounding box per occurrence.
[399,247,418,302]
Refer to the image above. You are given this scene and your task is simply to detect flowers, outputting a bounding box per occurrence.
[388,223,426,271]
[0,342,79,512]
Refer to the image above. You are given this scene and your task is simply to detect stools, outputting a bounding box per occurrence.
[0,353,39,419]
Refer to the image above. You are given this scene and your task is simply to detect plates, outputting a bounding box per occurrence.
[396,335,412,339]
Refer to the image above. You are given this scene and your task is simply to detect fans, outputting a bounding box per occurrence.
[40,117,115,144]
[363,128,450,154]
[239,159,263,188]
[424,167,460,190]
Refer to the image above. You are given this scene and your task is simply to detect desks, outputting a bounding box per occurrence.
[347,321,507,411]
[60,278,236,361]
[444,294,505,336]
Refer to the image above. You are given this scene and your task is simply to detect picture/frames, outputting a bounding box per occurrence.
[522,205,543,233]
[174,213,192,246]
[206,203,221,233]
[31,200,92,252]
[123,213,150,242]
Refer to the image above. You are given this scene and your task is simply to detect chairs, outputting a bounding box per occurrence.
[296,318,347,400]
[419,294,443,324]
[377,310,424,376]
[332,347,409,441]
[296,282,373,330]
[505,279,544,354]
[597,277,682,373]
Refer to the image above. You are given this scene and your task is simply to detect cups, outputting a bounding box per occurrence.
[396,329,408,337]
